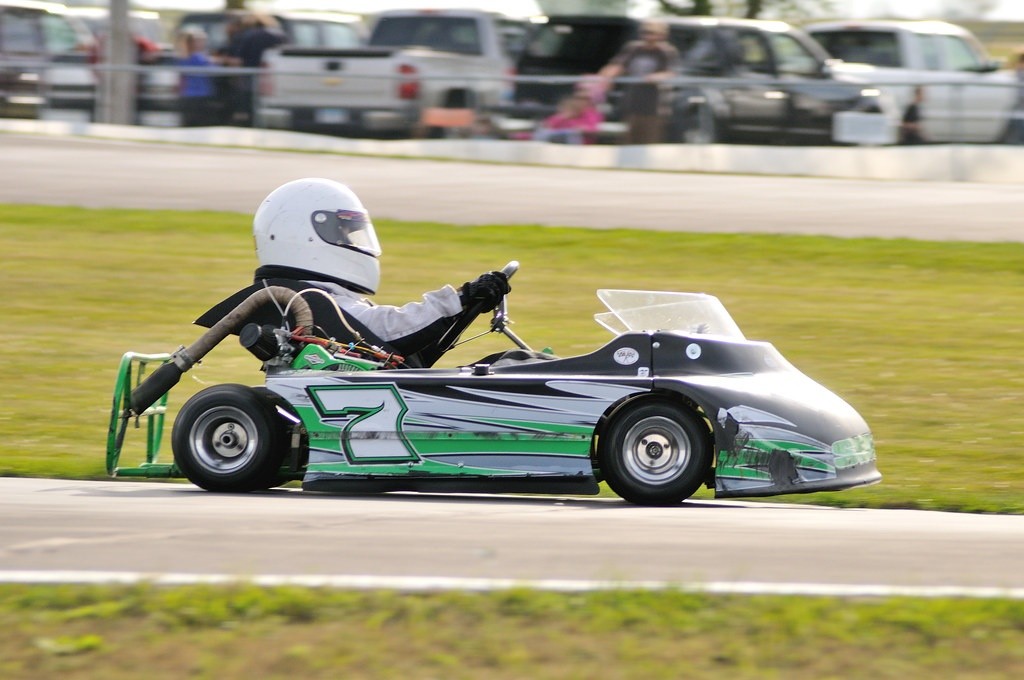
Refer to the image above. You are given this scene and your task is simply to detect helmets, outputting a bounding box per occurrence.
[253,178,382,296]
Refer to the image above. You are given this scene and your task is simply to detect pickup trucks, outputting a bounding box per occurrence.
[249,2,519,137]
[489,16,886,146]
[804,21,1023,145]
[39,8,374,127]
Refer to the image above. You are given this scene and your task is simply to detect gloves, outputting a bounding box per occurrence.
[455,270,512,313]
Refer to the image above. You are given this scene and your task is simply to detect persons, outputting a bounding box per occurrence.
[211,10,290,125]
[542,99,604,147]
[596,16,682,117]
[175,27,226,127]
[897,82,929,145]
[252,178,512,367]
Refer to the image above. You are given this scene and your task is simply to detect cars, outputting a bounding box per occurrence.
[0,1,103,83]
